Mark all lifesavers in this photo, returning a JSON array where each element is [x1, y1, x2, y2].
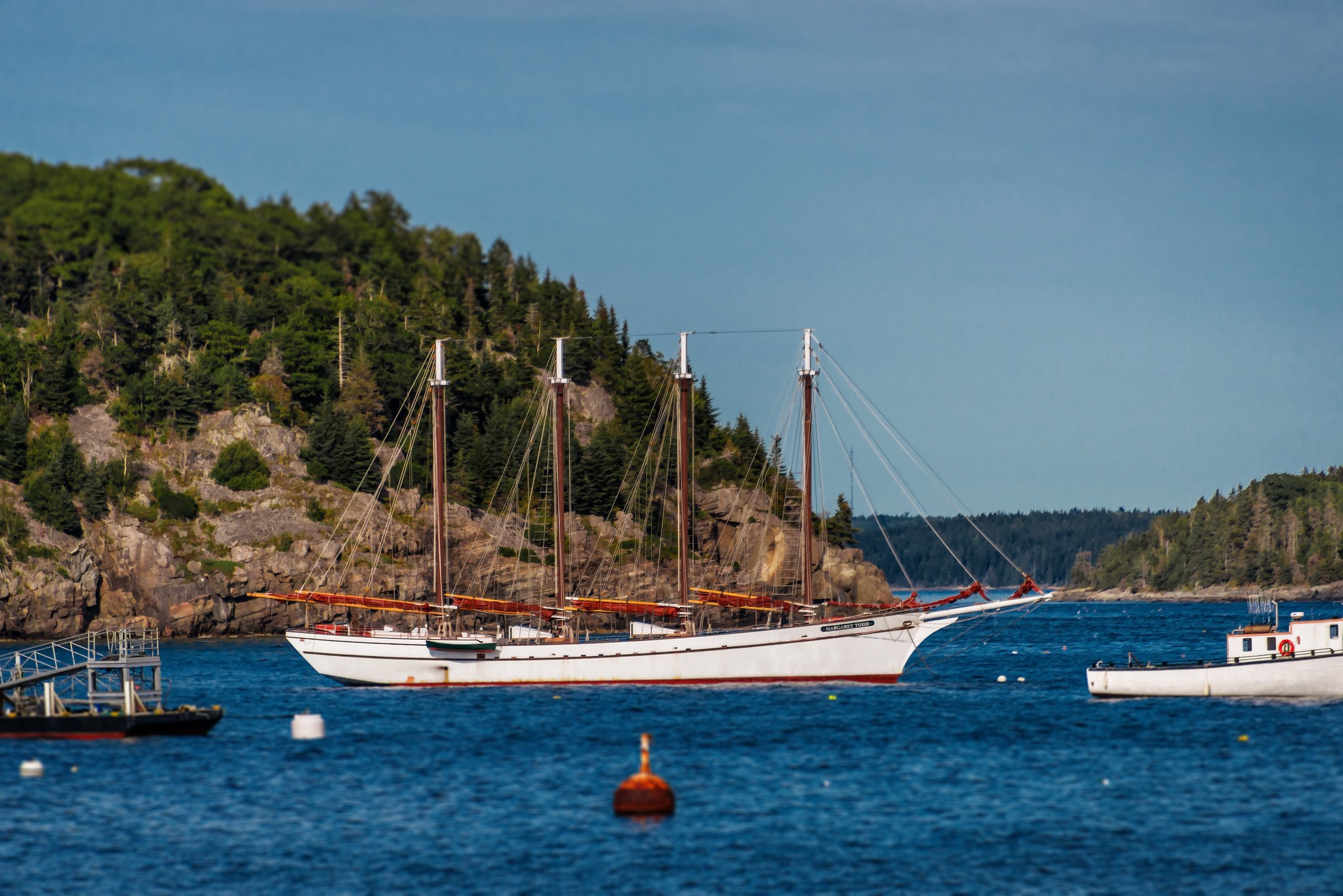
[[1278, 640, 1294, 656]]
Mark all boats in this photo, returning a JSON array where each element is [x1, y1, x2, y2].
[[1086, 600, 1342, 697], [425, 639, 497, 653], [0, 701, 226, 740]]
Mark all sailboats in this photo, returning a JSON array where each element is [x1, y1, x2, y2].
[[244, 329, 1057, 684]]
